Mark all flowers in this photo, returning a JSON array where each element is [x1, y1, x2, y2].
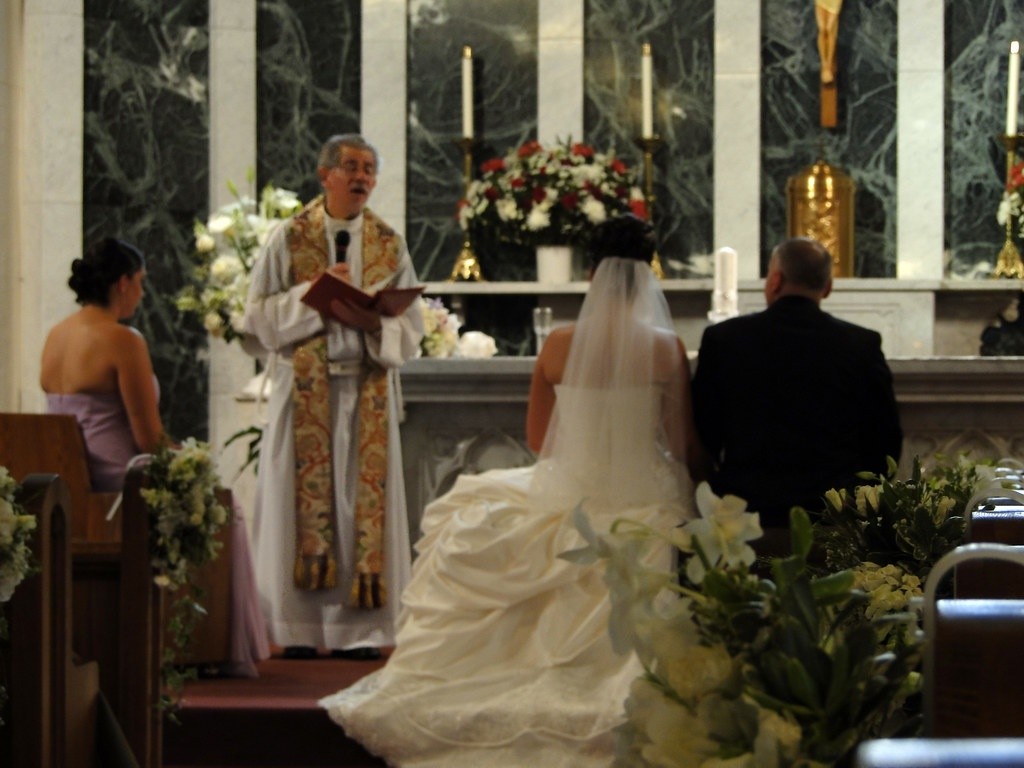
[[0, 461, 44, 647], [418, 299, 499, 357], [453, 134, 652, 263], [577, 449, 1020, 768], [170, 162, 303, 341], [138, 435, 232, 732]]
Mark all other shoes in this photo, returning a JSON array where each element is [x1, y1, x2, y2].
[[330, 648, 379, 662], [285, 647, 321, 660]]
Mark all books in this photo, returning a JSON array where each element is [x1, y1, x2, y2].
[[300, 273, 425, 337]]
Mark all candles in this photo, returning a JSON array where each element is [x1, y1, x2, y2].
[[461, 44, 474, 137], [1004, 40, 1019, 136], [713, 245, 736, 314], [640, 42, 652, 139]]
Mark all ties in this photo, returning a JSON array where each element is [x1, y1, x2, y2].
[[334, 229, 352, 265]]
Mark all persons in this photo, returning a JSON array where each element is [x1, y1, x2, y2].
[[39, 234, 267, 685], [251, 134, 429, 657], [318, 214, 713, 768], [689, 239, 903, 541]]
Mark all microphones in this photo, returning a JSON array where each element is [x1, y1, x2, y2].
[[334, 229, 350, 264]]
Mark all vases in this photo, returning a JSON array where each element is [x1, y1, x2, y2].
[[534, 246, 584, 283]]
